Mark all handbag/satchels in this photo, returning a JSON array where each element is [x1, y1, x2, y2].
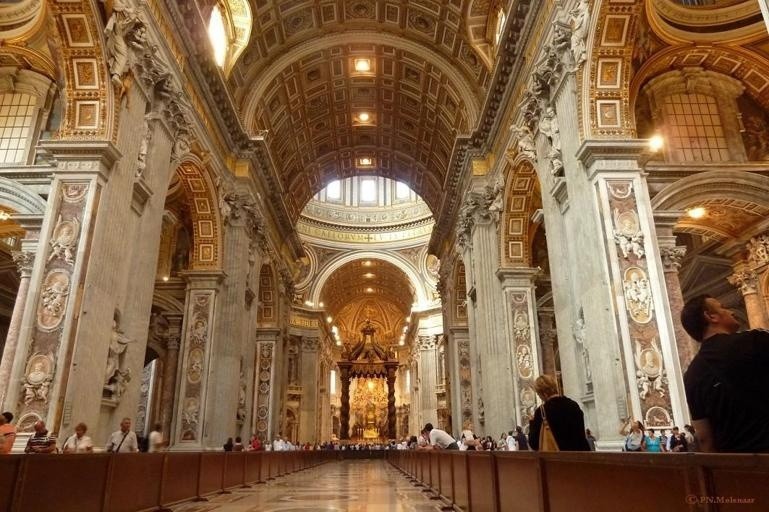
[[538, 404, 560, 452]]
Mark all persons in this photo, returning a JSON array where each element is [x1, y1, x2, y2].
[[220, 415, 694, 454], [528, 374, 591, 451], [679, 292, 768, 454], [24, 421, 58, 455], [42, 235, 76, 317], [0, 411, 17, 454], [612, 229, 633, 258], [104, 418, 140, 454], [21, 379, 50, 406], [62, 422, 93, 454], [146, 421, 169, 454], [653, 373, 666, 400], [507, 28, 577, 172], [636, 370, 652, 400], [628, 232, 646, 259]]
